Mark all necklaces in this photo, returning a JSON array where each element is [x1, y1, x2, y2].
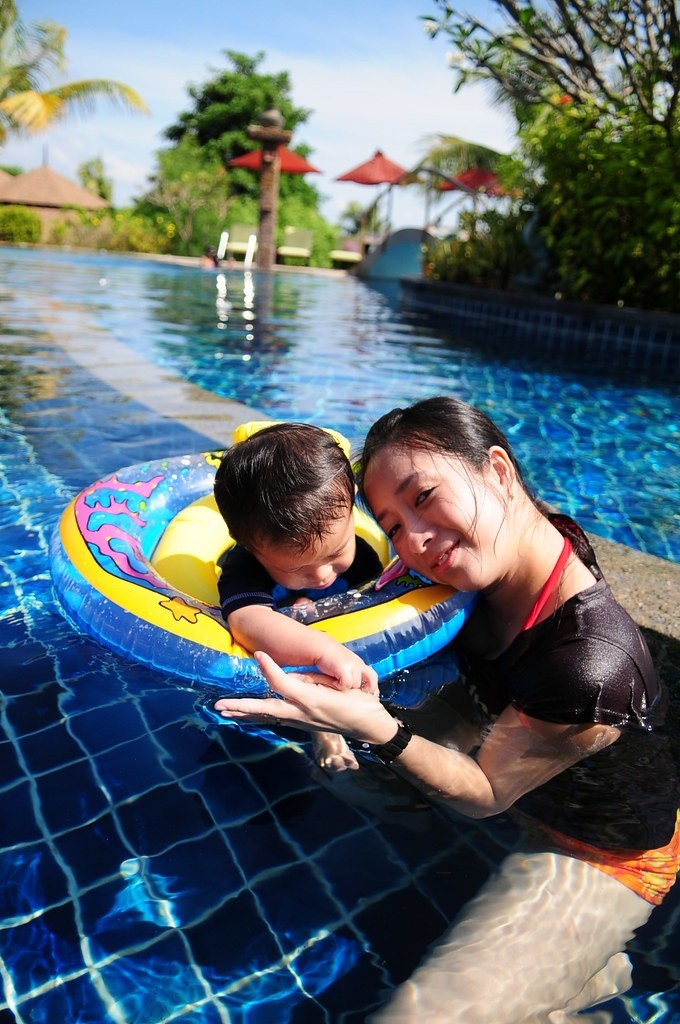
[[512, 538, 572, 637]]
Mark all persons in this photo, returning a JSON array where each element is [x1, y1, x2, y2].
[[215, 397, 680, 1024], [216, 423, 385, 772]]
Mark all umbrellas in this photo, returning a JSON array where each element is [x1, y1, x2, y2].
[[229, 142, 523, 239]]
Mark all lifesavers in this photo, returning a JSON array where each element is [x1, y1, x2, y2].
[[45, 446, 475, 689]]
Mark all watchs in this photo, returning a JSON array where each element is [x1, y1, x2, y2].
[[346, 700, 412, 764]]
